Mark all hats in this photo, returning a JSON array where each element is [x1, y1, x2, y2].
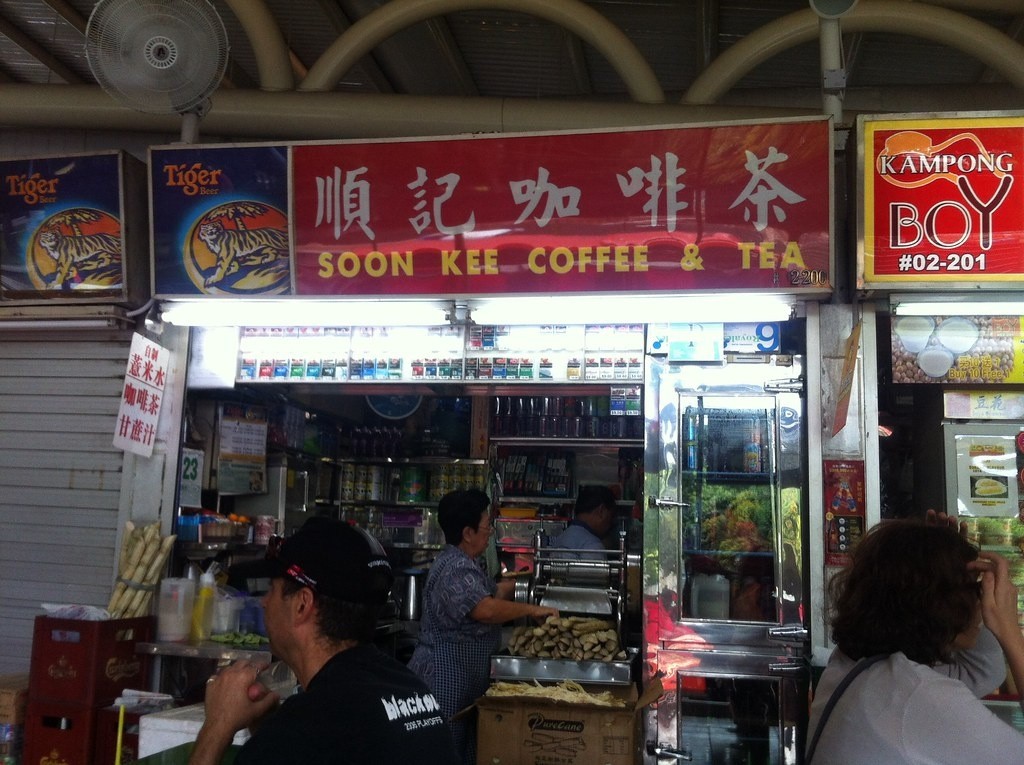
[[242, 517, 394, 608]]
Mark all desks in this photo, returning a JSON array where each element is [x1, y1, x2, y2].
[[138, 641, 274, 698]]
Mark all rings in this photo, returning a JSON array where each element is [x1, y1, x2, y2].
[[248, 663, 260, 675], [207, 678, 215, 684]]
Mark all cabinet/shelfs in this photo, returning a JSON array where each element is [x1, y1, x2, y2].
[[172, 389, 643, 574]]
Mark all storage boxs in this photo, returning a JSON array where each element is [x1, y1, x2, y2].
[[0, 611, 252, 765], [445, 676, 664, 765]]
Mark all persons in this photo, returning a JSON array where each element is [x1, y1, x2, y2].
[[805, 508, 1024, 765], [189, 517, 454, 765], [406, 489, 560, 765], [550, 485, 617, 561], [718, 541, 798, 765]]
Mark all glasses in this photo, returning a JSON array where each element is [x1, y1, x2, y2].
[[478, 517, 497, 531]]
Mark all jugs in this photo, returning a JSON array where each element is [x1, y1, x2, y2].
[[157, 578, 195, 643], [390, 574, 420, 621]]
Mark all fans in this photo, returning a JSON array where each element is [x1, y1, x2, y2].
[[366, 394, 424, 420], [85, 0, 231, 146]]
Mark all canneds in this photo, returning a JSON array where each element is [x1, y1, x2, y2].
[[683, 442, 698, 470], [743, 443, 761, 472], [492, 396, 633, 438], [683, 414, 697, 442], [341, 464, 484, 501]]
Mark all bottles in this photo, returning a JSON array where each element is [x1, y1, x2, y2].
[[201, 510, 231, 541]]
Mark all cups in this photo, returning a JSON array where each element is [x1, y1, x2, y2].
[[192, 588, 217, 641]]
